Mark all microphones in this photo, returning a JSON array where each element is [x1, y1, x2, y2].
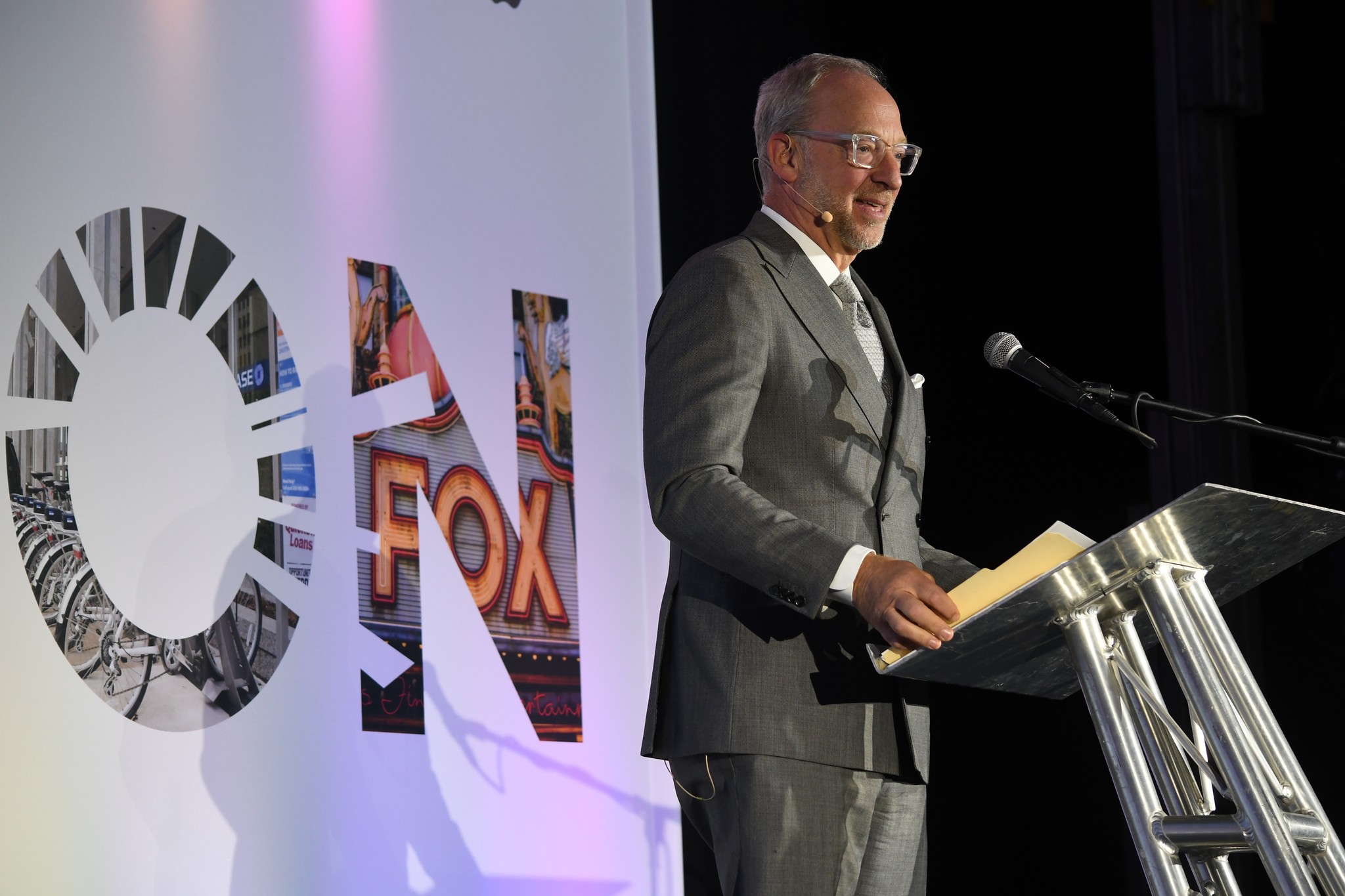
[[983, 331, 1120, 426], [779, 176, 834, 222]]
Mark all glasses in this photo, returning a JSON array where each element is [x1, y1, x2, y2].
[[786, 123, 922, 178]]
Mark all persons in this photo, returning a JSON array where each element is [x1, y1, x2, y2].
[[641, 53, 982, 896]]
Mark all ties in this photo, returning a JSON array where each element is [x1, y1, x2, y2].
[[831, 273, 884, 384]]
